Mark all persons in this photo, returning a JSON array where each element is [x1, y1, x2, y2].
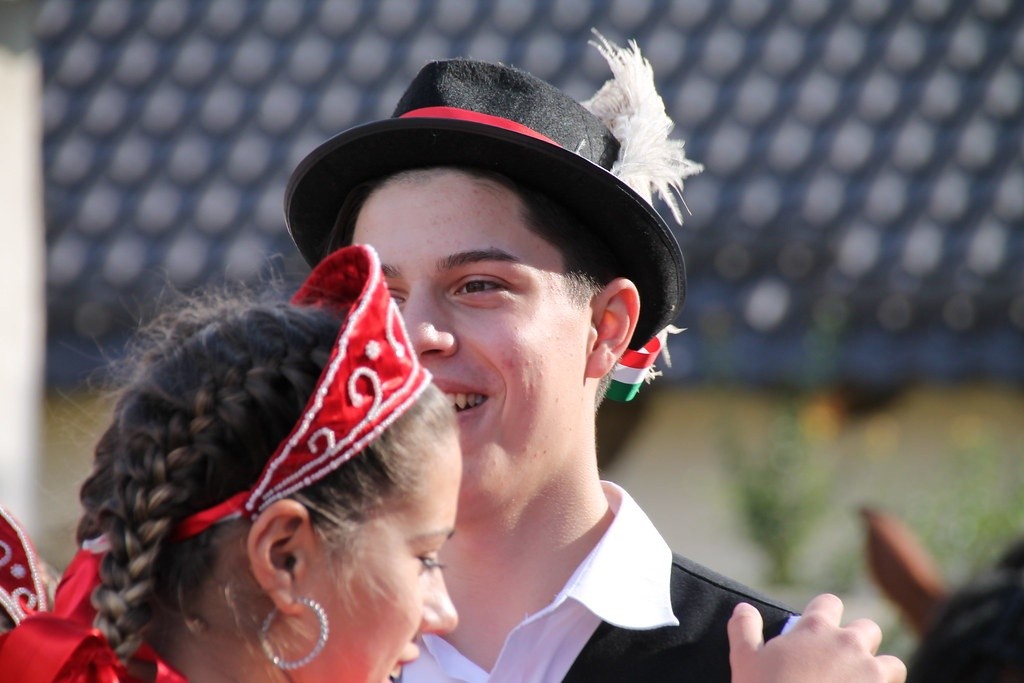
[[282, 57, 802, 683], [0, 246, 907, 683]]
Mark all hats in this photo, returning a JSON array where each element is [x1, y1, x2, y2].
[[284, 28, 703, 402]]
